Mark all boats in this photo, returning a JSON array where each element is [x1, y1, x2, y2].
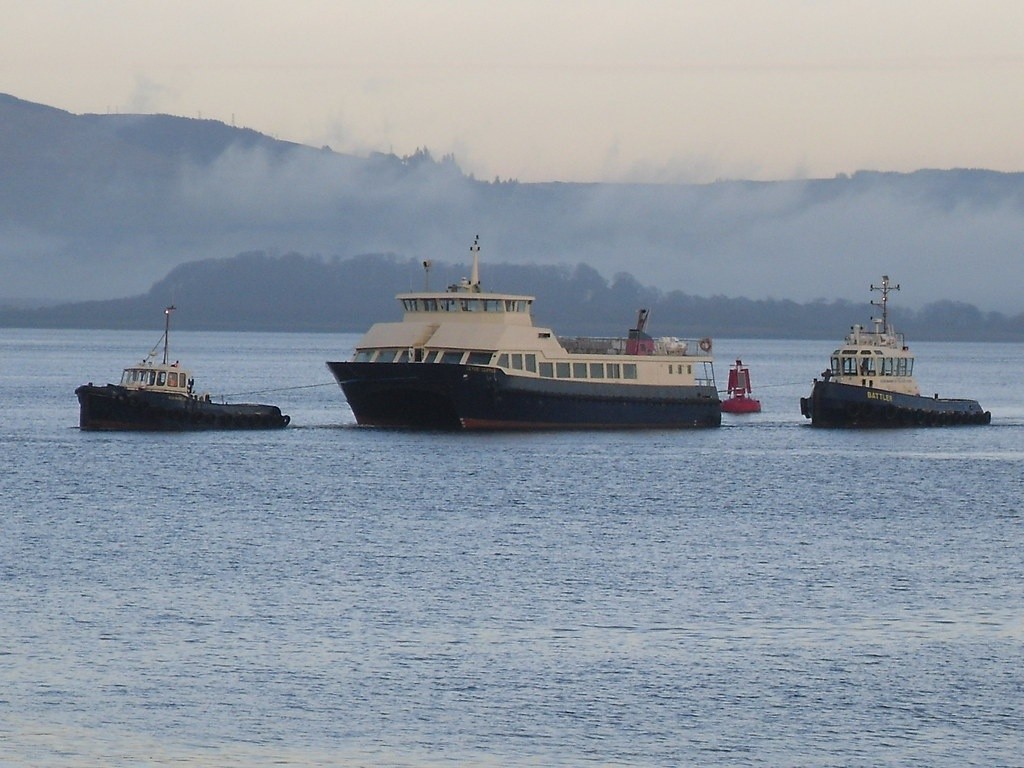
[[74, 304, 292, 431], [326, 233, 724, 430], [799, 275, 992, 428]]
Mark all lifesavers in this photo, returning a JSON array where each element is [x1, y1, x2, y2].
[[135, 400, 292, 427], [700, 339, 712, 351], [884, 404, 993, 426]]
